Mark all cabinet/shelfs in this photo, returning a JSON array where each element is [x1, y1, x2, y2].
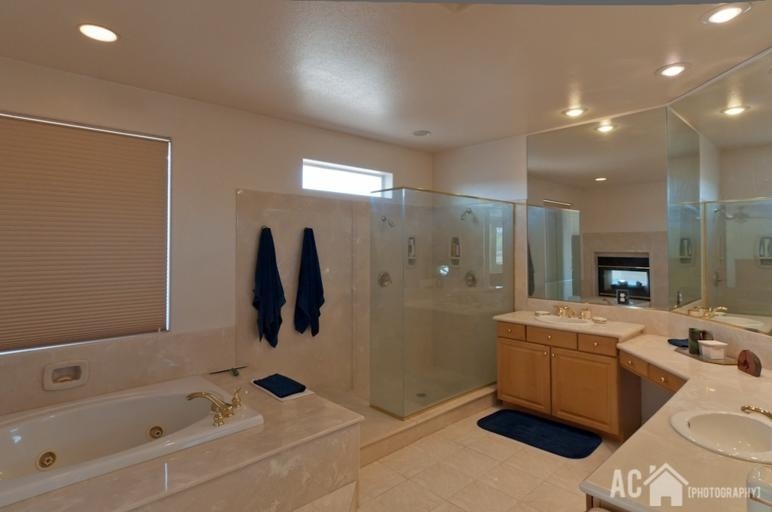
[[617, 332, 753, 396], [492, 308, 645, 445], [579, 378, 772, 512]]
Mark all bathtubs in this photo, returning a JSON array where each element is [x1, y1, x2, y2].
[[0, 375, 265, 509]]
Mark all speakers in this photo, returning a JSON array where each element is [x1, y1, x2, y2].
[[616, 290, 629, 304]]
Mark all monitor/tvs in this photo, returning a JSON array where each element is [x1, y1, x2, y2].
[[598, 266, 650, 301]]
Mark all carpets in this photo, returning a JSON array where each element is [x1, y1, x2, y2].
[[477, 408, 602, 459]]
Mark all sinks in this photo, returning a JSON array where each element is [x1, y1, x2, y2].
[[535, 316, 593, 326], [669, 410, 772, 464]]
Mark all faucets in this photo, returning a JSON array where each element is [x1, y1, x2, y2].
[[185, 385, 245, 425], [741, 404, 772, 422], [455, 204, 477, 226], [552, 303, 571, 318]]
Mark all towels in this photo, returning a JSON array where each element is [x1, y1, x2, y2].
[[253, 374, 306, 398], [250, 226, 287, 350], [293, 225, 325, 338]]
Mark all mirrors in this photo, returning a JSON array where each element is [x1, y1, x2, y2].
[[527, 45, 772, 341]]
